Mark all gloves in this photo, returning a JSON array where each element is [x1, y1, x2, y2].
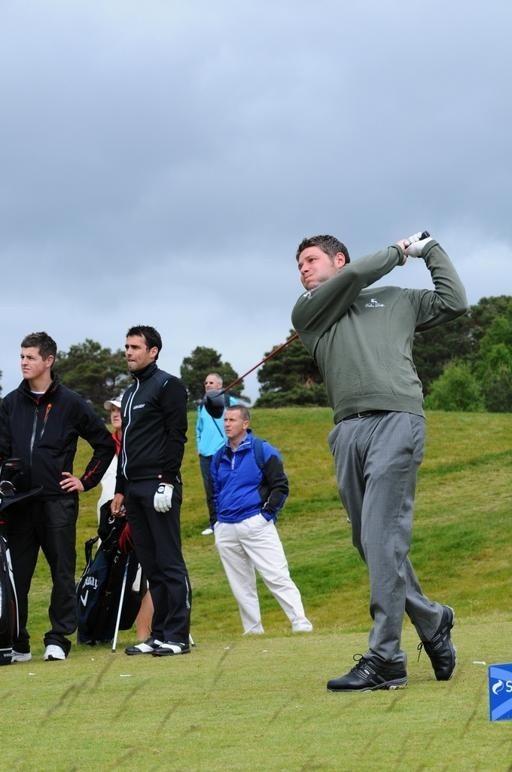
[[402, 230, 436, 259], [152, 481, 175, 513]]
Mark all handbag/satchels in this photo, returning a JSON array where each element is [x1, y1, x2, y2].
[[0, 533, 21, 668], [75, 498, 150, 647]]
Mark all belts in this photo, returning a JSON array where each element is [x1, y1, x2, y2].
[[339, 410, 387, 422]]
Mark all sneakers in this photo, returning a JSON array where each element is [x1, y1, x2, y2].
[[125, 637, 166, 655], [326, 654, 410, 693], [40, 642, 66, 662], [151, 640, 190, 656], [201, 525, 215, 536], [6, 647, 34, 663], [421, 604, 456, 682]]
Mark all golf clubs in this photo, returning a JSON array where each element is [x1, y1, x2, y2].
[[204, 229, 427, 418]]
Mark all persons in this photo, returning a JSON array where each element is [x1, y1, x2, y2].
[[109, 323, 193, 657], [194, 371, 240, 536], [0, 330, 118, 664], [98, 389, 155, 643], [205, 404, 316, 635], [290, 232, 470, 693]]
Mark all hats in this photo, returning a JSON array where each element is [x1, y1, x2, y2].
[[102, 393, 124, 411]]
